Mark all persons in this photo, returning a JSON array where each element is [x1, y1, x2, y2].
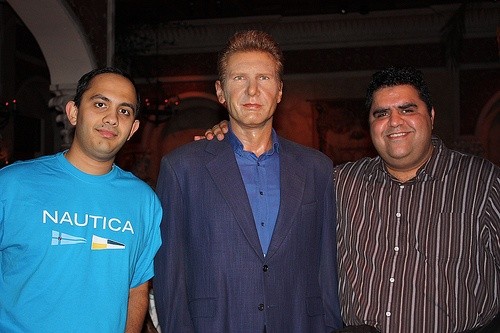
[[151, 29, 345, 333], [193, 69, 500, 333], [0, 67, 163, 333]]
